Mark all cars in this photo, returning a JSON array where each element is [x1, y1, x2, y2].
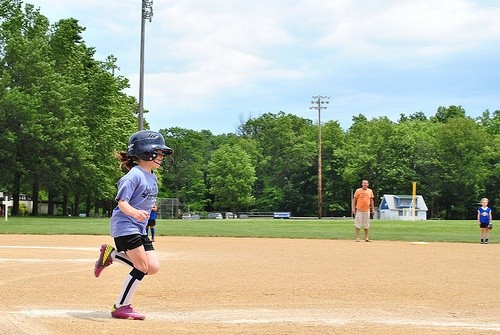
[[182, 212, 200, 220], [228, 213, 237, 219], [213, 213, 223, 220]]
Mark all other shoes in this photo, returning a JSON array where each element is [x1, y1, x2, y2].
[[480, 239, 483, 244], [365, 239, 369, 241], [485, 239, 488, 244]]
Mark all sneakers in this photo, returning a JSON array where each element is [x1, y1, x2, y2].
[[94, 244, 115, 277], [111, 303, 145, 320]]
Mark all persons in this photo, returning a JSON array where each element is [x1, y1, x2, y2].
[[146, 203, 157, 242], [94, 131, 173, 320], [352, 180, 375, 242], [477, 198, 492, 244]]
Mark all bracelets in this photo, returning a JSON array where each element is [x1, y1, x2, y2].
[[372, 209, 374, 211]]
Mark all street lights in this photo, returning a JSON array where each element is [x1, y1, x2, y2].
[[310, 94, 330, 219], [137, 0, 155, 131]]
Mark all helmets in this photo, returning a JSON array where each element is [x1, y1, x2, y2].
[[128, 130, 174, 161]]
